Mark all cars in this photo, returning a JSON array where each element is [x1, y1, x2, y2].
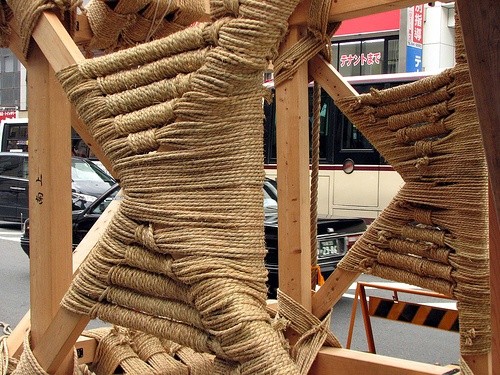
[[1, 150, 120, 227], [23, 178, 367, 302]]
